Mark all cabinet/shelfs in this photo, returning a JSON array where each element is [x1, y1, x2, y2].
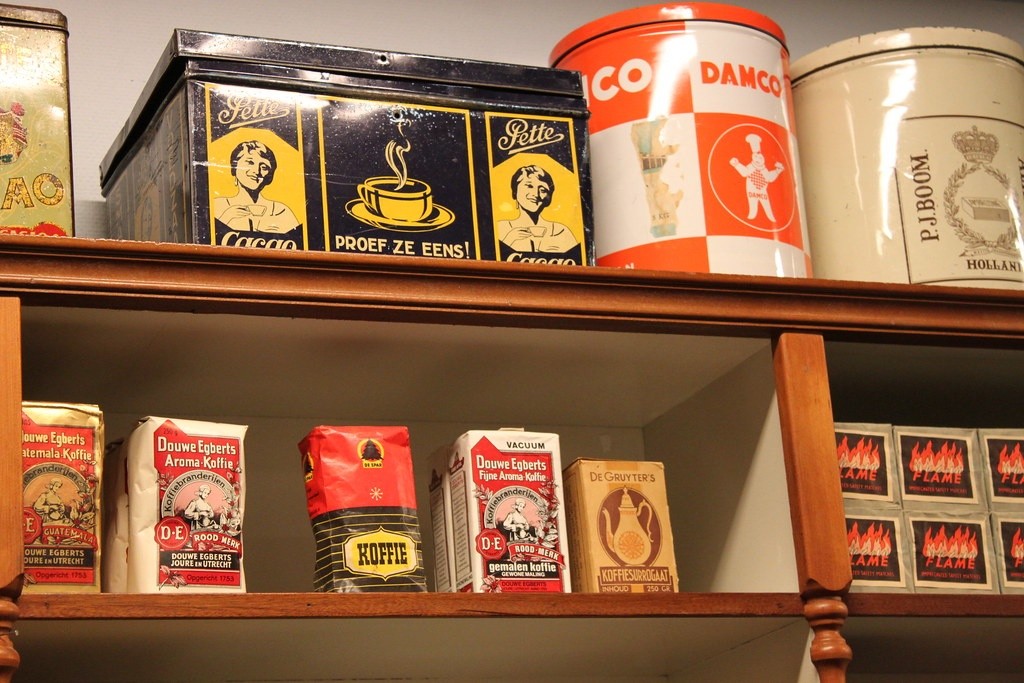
[[0, 232, 1024, 683]]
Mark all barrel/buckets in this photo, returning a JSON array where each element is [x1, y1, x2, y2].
[[789, 27, 1024, 291], [549, 2, 813, 279]]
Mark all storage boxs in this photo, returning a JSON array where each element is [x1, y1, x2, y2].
[[101, 435, 129, 592], [298, 424, 428, 592], [990, 512, 1024, 595], [892, 425, 988, 512], [100, 28, 594, 263], [0, 4, 77, 237], [426, 460, 457, 593], [126, 415, 250, 593], [833, 421, 903, 510], [844, 508, 915, 595], [445, 428, 573, 594], [977, 428, 1024, 512], [21, 399, 107, 595], [561, 457, 681, 593], [904, 512, 1001, 596]]
